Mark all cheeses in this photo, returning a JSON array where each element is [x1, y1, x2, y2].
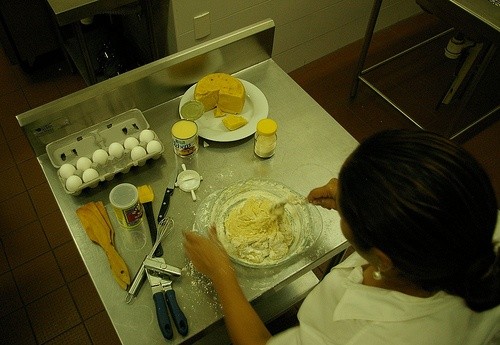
[[194, 73, 248, 130]]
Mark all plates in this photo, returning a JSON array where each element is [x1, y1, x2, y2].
[[178, 78, 269, 142]]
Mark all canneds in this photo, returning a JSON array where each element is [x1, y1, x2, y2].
[[109, 183, 144, 229], [171, 100, 204, 158]]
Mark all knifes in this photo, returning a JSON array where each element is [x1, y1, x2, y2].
[[157, 168, 178, 225]]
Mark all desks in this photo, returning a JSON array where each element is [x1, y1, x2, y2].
[[36, 57, 360, 345], [47, 0, 160, 86], [351, 0, 500, 142]]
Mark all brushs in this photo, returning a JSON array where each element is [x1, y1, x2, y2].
[[138, 185, 164, 257]]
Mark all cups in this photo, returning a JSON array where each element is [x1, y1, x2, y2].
[[109, 183, 144, 228], [171, 119, 199, 159]]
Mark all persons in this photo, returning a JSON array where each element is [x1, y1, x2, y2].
[[182, 129, 499, 343]]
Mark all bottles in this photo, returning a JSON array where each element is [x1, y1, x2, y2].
[[253, 118, 278, 159]]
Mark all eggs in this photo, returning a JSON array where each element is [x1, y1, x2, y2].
[[59, 129, 162, 191]]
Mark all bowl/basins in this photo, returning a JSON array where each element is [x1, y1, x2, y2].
[[191, 177, 323, 269]]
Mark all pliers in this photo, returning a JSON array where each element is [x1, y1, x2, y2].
[[144, 258, 188, 339]]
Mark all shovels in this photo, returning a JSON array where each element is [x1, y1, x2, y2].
[[75, 201, 131, 288]]
